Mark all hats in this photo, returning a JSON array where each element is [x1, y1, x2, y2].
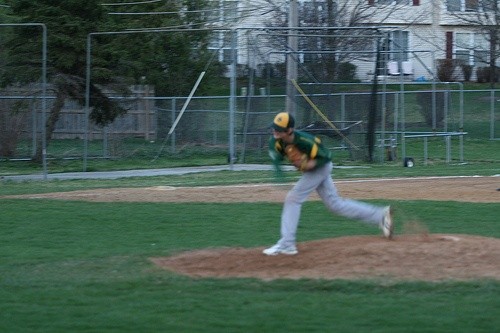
[[266, 111, 296, 132]]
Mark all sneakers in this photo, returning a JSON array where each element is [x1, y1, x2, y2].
[[261, 238, 299, 256], [379, 204, 394, 239]]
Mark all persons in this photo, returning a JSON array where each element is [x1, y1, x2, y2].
[[261, 112, 392, 256]]
